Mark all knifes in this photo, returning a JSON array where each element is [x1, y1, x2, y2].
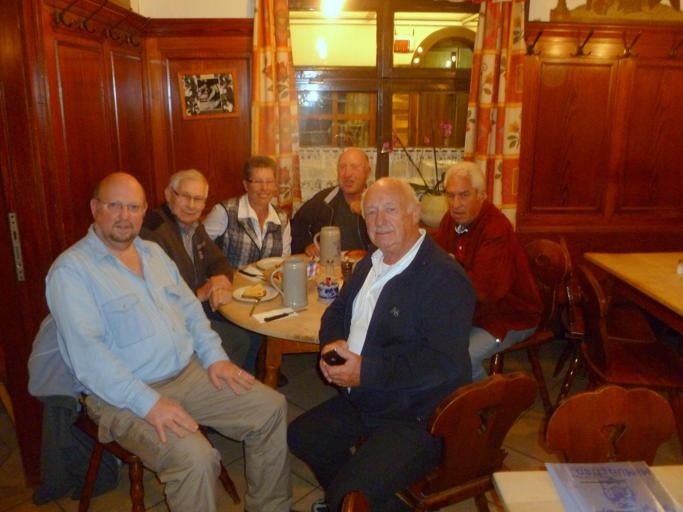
[[263, 307, 308, 324]]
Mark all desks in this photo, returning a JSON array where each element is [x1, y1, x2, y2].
[[492, 465, 682, 512], [585, 250, 683, 344]]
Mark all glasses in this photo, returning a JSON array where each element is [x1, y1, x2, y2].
[[95, 196, 146, 214], [247, 178, 275, 186], [169, 185, 207, 202]]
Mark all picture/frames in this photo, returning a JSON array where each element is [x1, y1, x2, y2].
[[176, 69, 243, 121]]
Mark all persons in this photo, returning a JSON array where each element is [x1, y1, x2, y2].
[[45, 172, 292, 512], [431, 162, 547, 380], [141, 169, 252, 368], [291, 147, 379, 257], [202, 155, 292, 388], [287, 176, 475, 512]]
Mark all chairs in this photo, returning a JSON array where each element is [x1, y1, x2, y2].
[[398, 372, 542, 512], [555, 236, 596, 375], [559, 265, 679, 394], [75, 413, 242, 512], [490, 241, 560, 375], [545, 385, 678, 466]]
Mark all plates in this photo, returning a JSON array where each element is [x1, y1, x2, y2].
[[256, 256, 282, 272], [230, 283, 280, 302]]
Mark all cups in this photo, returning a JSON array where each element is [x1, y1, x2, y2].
[[313, 226, 342, 266], [270, 255, 307, 308]]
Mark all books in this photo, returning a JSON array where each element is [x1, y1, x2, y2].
[[542, 462, 681, 508]]
